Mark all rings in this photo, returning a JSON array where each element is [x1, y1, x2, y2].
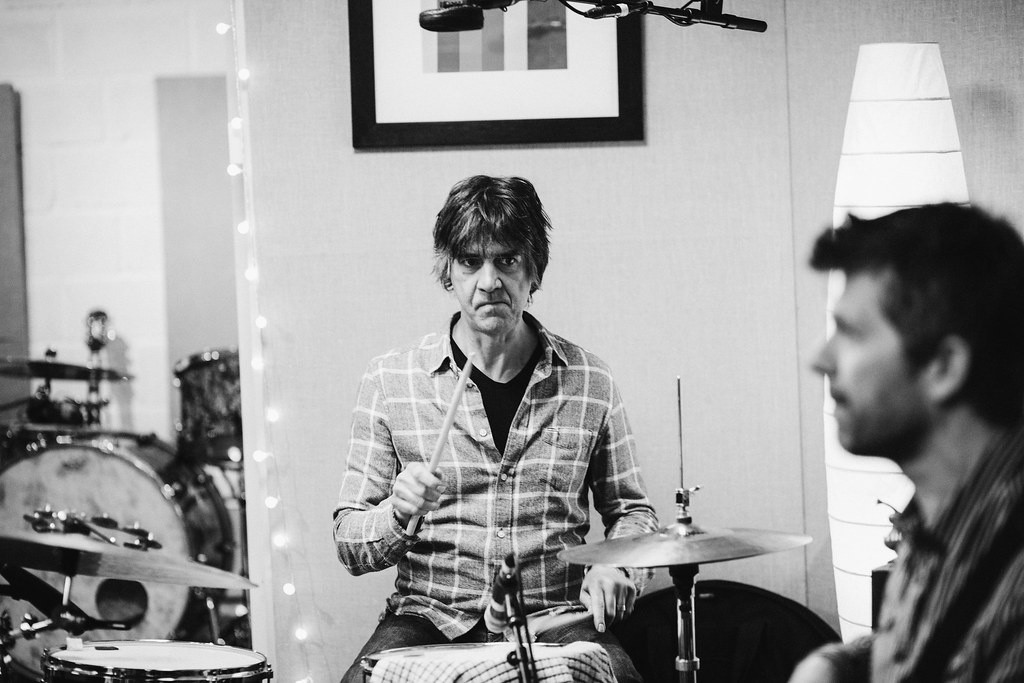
[[617, 603, 626, 611]]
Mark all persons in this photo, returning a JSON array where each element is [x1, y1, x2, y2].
[[331, 173, 662, 683], [786, 199, 1023, 683]]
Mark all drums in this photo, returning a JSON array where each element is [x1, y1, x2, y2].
[[174, 344, 240, 460], [0, 428, 235, 680], [362, 642, 611, 683], [38, 639, 274, 683]]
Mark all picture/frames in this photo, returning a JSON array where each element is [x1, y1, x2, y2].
[[347, 0, 644, 149]]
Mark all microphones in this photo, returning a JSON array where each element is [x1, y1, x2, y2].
[[484, 553, 516, 634], [419, 0, 518, 32]]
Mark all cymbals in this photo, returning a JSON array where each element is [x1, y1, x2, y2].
[[0, 357, 134, 382], [558, 522, 814, 570], [0, 537, 260, 589]]
